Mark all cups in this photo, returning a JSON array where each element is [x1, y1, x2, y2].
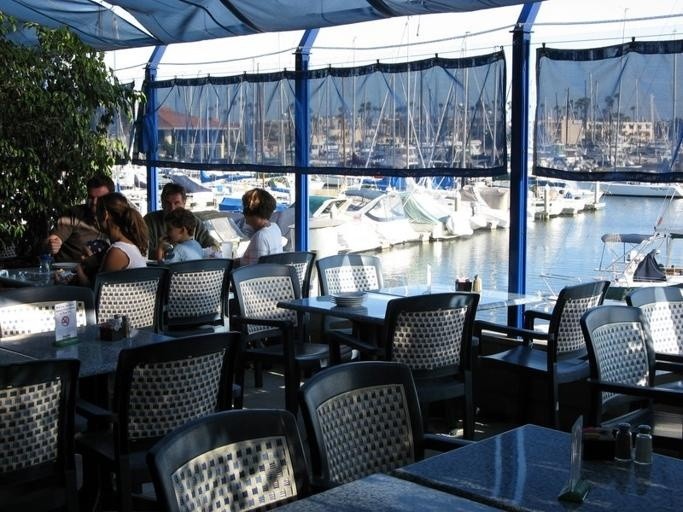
[[232, 236, 239, 250], [221, 242, 232, 259]]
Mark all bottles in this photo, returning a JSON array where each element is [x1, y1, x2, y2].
[[614, 423, 632, 461], [454, 274, 481, 292], [579, 427, 618, 463], [97, 314, 130, 341]]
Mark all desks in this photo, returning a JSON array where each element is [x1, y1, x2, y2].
[[393, 423, 683, 512]]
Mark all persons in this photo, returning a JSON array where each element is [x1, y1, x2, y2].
[[42, 174, 284, 315]]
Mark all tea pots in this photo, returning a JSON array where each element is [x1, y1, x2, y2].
[[36, 254, 50, 284]]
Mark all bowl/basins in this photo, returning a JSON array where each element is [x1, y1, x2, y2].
[[52, 262, 77, 269], [18, 271, 55, 280], [330, 292, 367, 306]]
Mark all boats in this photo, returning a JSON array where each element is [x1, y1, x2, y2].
[[536, 184, 682, 320]]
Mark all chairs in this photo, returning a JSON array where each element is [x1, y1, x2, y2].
[[0, 250, 499, 512], [469, 280, 683, 461]]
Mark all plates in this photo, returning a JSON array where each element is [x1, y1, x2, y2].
[[633, 424, 654, 465]]
